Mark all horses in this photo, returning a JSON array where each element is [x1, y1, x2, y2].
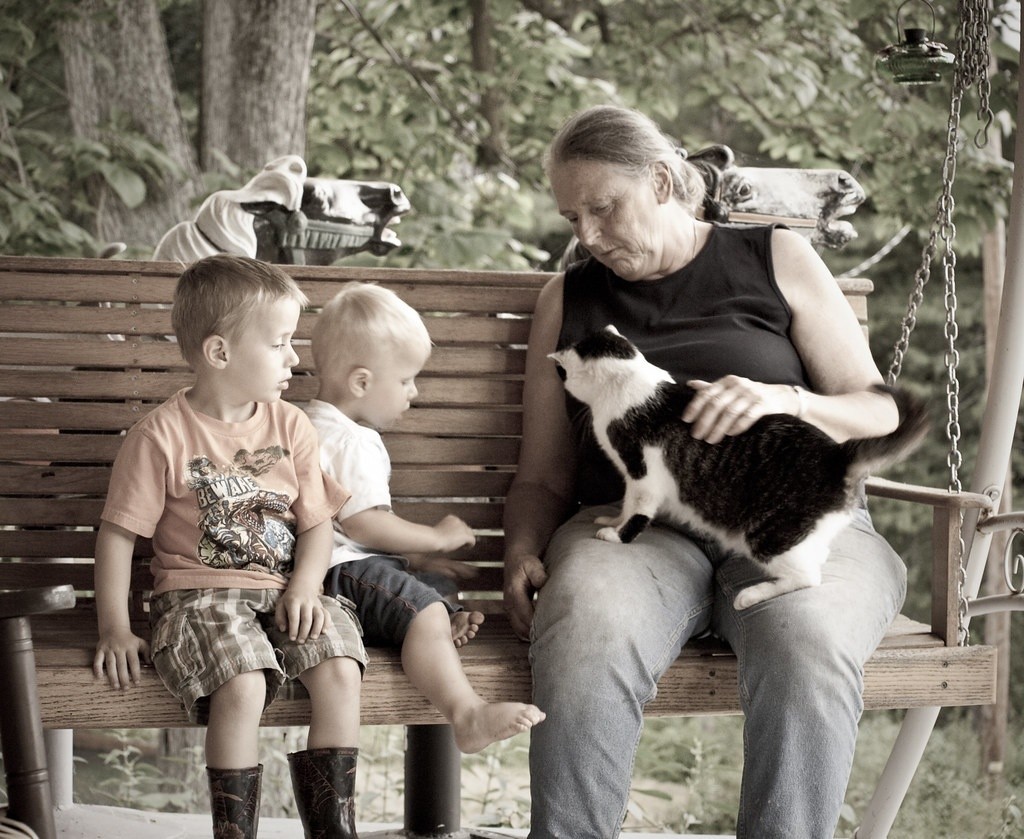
[[556, 143, 867, 272]]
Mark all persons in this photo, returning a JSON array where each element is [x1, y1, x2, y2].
[[503, 103, 908, 837], [95, 255, 368, 839], [303, 283, 546, 754]]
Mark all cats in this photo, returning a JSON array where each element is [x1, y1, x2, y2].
[[545, 322, 935, 613]]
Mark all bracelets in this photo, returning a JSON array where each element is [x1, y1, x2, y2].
[[790, 381, 808, 419]]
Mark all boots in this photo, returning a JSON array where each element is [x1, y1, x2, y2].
[[203, 763, 262, 838], [286, 747, 359, 839]]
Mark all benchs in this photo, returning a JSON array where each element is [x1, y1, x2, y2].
[[0, 250, 997, 839]]
